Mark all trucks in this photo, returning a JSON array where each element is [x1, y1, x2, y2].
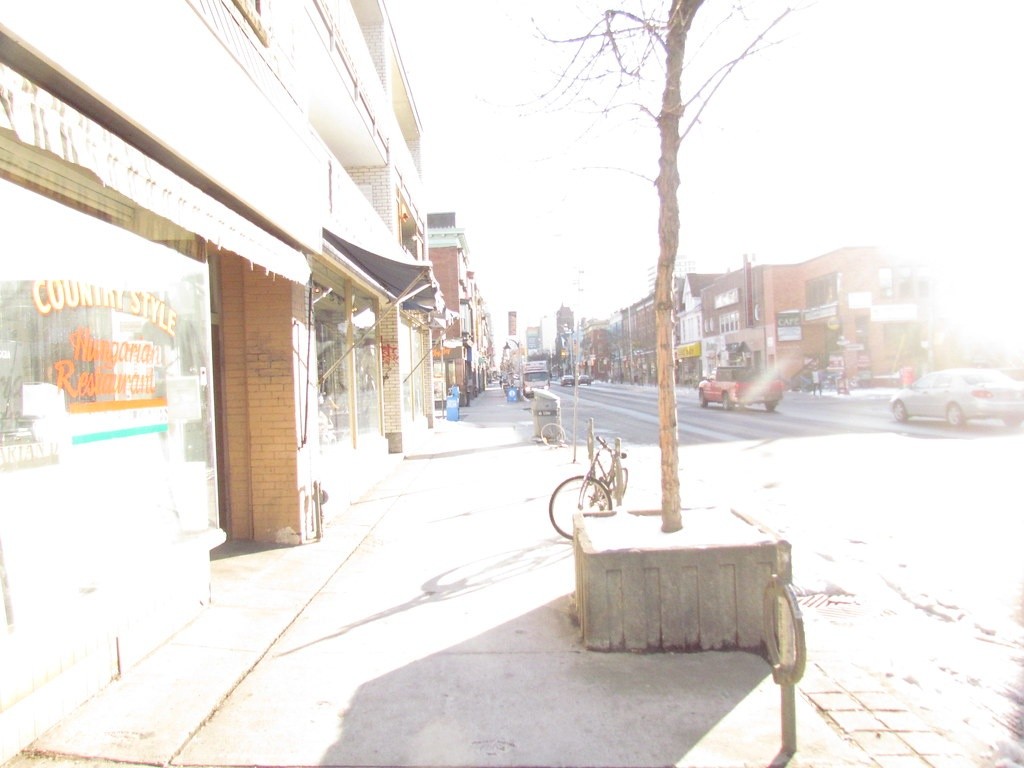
[[521, 361, 553, 398]]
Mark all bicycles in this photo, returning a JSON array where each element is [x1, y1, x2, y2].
[[548, 435, 629, 540], [536, 423, 568, 449]]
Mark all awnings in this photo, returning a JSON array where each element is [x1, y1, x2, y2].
[[318, 229, 458, 384]]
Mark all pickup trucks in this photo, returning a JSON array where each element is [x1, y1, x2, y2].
[[698, 366, 783, 411]]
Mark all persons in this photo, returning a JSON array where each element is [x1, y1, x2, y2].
[[899, 363, 912, 385], [811, 367, 823, 395]]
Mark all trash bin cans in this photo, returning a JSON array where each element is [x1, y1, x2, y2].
[[531, 387, 561, 439], [446, 395, 459, 421], [506, 387, 518, 401]]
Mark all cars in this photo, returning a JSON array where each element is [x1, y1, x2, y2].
[[891, 367, 1024, 427], [513, 374, 519, 380], [578, 375, 591, 386], [560, 375, 575, 387], [782, 373, 814, 393]]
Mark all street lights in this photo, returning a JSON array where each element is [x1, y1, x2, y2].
[[540, 316, 547, 360], [623, 306, 634, 385]]
